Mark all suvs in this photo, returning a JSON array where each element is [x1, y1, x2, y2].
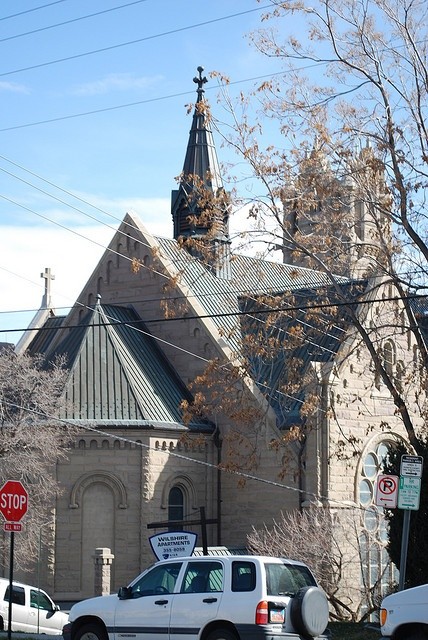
[[380, 584, 428, 640], [68, 556, 329, 640], [0, 580, 68, 635]]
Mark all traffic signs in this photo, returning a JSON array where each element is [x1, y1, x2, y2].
[[398, 477, 421, 510], [400, 454, 423, 477]]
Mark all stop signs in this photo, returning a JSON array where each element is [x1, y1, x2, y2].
[[0, 481, 28, 522]]
